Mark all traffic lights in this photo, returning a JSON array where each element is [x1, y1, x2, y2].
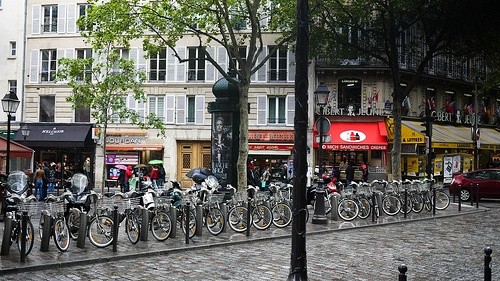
[[420, 116, 429, 137]]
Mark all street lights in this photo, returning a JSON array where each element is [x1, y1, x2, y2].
[[2, 91, 20, 176], [312, 78, 331, 223]]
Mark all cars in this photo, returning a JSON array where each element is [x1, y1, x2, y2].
[[449, 169, 500, 203]]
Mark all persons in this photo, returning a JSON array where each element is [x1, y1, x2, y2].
[[33, 163, 45, 200], [345, 163, 354, 182], [362, 164, 369, 182], [150, 164, 158, 189], [41, 161, 50, 197], [247, 156, 258, 197], [117, 169, 125, 192], [339, 157, 348, 169], [158, 163, 165, 186]]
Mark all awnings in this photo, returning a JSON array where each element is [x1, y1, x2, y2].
[[13, 122, 93, 147], [312, 117, 499, 152]]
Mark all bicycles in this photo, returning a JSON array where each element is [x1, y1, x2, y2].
[[38, 193, 71, 252], [253, 184, 293, 228], [285, 184, 310, 224], [193, 185, 225, 236], [132, 186, 173, 242], [159, 182, 198, 239], [312, 178, 449, 221], [65, 192, 115, 248], [212, 183, 253, 232], [100, 191, 141, 245], [229, 184, 273, 231], [8, 193, 34, 254]]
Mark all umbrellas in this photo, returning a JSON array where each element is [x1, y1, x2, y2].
[[134, 163, 149, 168], [185, 167, 215, 177], [115, 163, 127, 169], [148, 159, 164, 164]]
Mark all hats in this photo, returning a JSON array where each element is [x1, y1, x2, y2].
[[246, 156, 368, 173]]
